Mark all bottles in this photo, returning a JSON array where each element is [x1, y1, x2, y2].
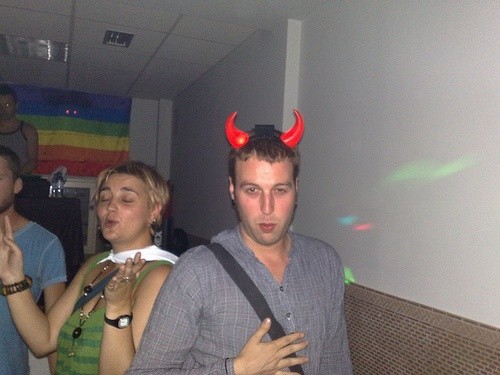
[[52, 172, 63, 198]]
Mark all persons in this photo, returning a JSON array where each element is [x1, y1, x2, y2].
[[0, 162, 179, 375], [0, 145, 67, 375], [124, 108, 354, 375], [0, 85, 39, 217]]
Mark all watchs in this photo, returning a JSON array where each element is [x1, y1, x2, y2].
[[103, 313, 133, 328]]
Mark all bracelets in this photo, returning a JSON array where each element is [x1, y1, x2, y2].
[[0, 274, 34, 295]]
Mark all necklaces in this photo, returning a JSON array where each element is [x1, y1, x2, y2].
[[67, 262, 113, 357]]
